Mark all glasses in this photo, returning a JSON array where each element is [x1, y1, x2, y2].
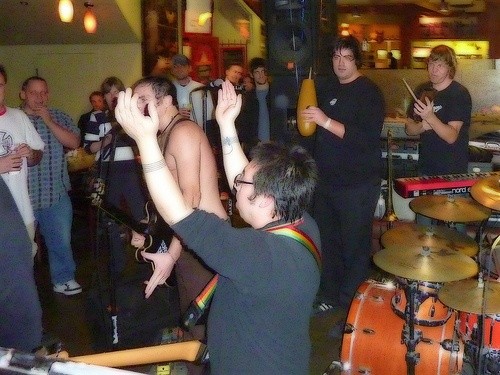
[[233, 174, 254, 185], [333, 55, 356, 62]]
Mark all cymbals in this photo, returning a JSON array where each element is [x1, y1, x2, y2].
[[470, 175, 500, 213], [380, 223, 479, 257], [436, 278, 500, 315], [372, 243, 479, 283], [409, 194, 492, 223]]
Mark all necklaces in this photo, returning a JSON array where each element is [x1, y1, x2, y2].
[[160, 112, 181, 135]]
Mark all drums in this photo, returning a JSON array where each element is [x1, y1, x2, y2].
[[475, 244, 500, 280], [454, 310, 500, 363], [338, 269, 471, 375]]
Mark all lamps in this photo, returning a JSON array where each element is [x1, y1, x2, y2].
[[57, 0, 75, 24], [81, 0, 99, 35]]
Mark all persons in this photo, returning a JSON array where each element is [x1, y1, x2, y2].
[[301, 34, 385, 313], [20, 76, 82, 294], [0, 174, 41, 352], [115, 80, 322, 375], [404, 45, 472, 234], [79, 56, 288, 338], [0, 65, 39, 257]]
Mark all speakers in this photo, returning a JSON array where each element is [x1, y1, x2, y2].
[[192, 78, 223, 91], [269, 74, 342, 155], [265, 0, 340, 74]]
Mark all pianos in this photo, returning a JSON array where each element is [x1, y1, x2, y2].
[[392, 171, 500, 231]]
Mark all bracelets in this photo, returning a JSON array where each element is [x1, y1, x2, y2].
[[167, 250, 177, 264], [324, 118, 331, 129]]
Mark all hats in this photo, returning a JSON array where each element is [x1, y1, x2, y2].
[[172, 54, 188, 64]]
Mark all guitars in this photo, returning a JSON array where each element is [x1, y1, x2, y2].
[[83, 170, 178, 291], [30, 332, 210, 369]]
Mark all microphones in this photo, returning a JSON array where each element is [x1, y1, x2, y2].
[[235, 85, 246, 91]]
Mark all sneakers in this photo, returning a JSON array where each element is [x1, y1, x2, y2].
[[314, 302, 337, 313], [329, 320, 346, 338], [53, 278, 82, 295]]
